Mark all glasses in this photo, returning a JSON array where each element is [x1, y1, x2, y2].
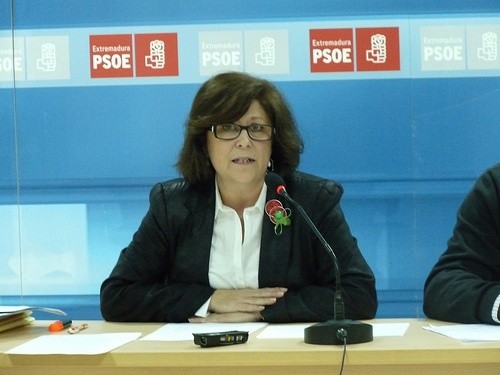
[[205, 122, 276, 141]]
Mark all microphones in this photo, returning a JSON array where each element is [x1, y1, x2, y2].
[[265, 172, 344, 323]]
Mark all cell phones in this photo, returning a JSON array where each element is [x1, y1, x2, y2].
[[192, 330, 248, 347]]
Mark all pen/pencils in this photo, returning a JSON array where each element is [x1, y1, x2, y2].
[[49, 318, 72, 333], [68, 324, 88, 334]]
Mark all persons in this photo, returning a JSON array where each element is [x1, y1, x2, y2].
[[423, 162, 500, 325], [99, 72, 378, 323]]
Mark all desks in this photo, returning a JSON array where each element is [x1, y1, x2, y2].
[[0, 319, 500, 375]]
